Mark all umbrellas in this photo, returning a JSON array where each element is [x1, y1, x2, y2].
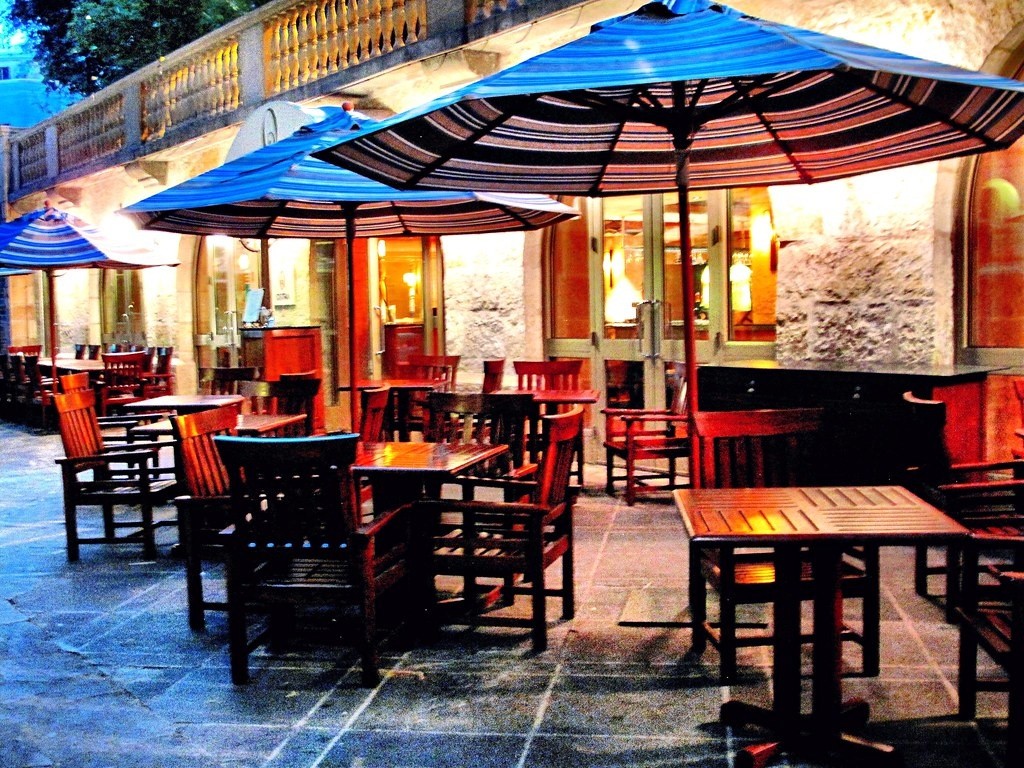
[[113, 102, 582, 432], [0, 201, 182, 396], [311, 0, 1024, 496]]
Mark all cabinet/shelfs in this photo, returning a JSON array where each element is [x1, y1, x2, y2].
[[240, 325, 327, 435], [382, 323, 423, 380]]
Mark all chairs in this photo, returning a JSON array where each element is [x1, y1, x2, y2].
[[0, 343, 1024, 768]]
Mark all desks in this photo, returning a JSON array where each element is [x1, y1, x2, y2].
[[490, 390, 602, 463], [338, 378, 451, 443], [123, 395, 244, 479], [351, 443, 509, 651], [37, 359, 139, 381], [668, 488, 972, 768]]
